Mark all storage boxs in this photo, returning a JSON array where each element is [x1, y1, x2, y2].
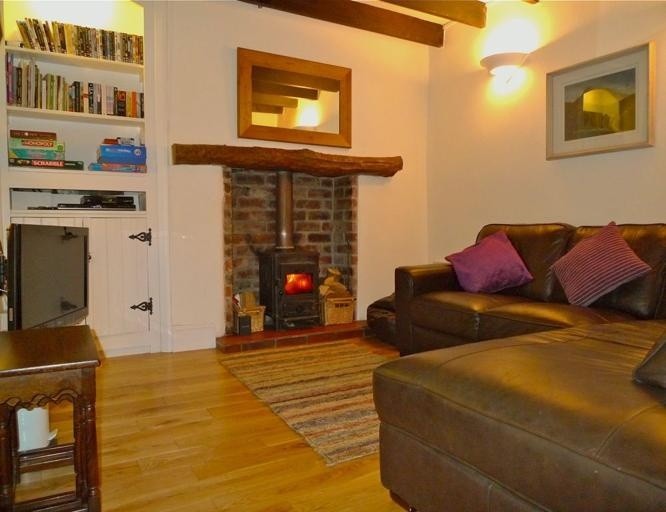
[[321, 295, 356, 325]]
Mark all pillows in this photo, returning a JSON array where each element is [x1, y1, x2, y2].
[[549, 221, 652, 308], [563, 223, 665, 319], [474, 223, 574, 302], [632, 329, 665, 389], [443, 225, 532, 292]]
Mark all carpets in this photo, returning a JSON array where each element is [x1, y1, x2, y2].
[[217, 338, 396, 468]]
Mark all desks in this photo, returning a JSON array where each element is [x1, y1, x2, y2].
[[0, 324, 103, 511]]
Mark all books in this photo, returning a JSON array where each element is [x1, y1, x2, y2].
[[2, 16, 147, 213]]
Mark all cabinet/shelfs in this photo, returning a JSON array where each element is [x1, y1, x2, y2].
[[0, 0, 152, 213], [0, 213, 152, 357]]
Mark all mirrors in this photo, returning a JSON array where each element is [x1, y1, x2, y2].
[[236, 46, 353, 149]]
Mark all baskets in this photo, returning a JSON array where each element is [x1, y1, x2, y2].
[[233, 305, 266, 335], [318, 297, 357, 326]]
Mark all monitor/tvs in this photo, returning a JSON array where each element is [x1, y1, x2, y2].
[[8, 224, 89, 329]]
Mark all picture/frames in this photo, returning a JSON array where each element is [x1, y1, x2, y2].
[[545, 40, 657, 161]]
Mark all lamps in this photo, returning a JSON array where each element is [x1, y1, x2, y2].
[[479, 52, 529, 76]]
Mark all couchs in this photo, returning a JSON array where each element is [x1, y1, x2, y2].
[[372, 220, 666, 512]]
